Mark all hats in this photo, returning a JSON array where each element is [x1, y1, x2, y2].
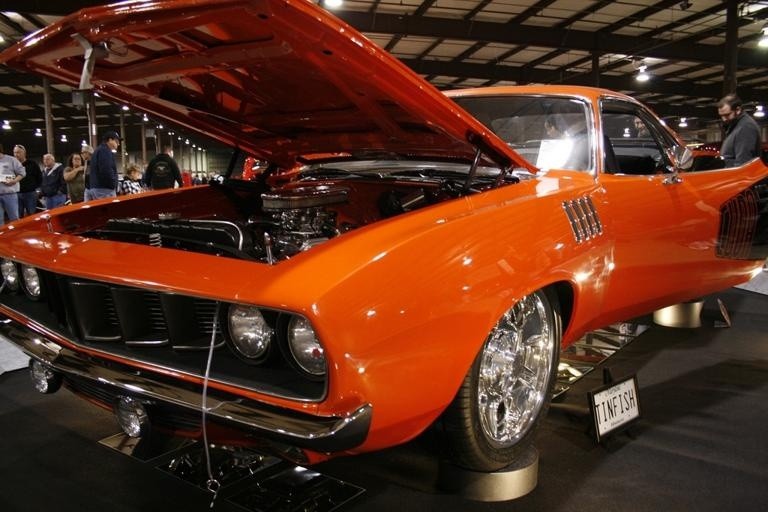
[[129, 165, 143, 174], [105, 131, 124, 141], [82, 145, 93, 154]]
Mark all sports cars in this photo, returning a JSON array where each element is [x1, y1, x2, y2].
[[0, 2, 767, 473]]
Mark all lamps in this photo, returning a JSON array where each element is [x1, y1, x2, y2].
[[753, 106, 765, 117], [758, 22, 768, 47], [635, 62, 650, 81], [1, 88, 207, 156]]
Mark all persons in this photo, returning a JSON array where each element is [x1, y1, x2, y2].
[[89, 131, 124, 200], [145, 140, 183, 191], [544, 114, 572, 139], [0, 144, 224, 226], [715, 93, 762, 167], [632, 114, 653, 139]]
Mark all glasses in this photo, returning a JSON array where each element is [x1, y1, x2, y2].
[[15, 145, 24, 150]]
